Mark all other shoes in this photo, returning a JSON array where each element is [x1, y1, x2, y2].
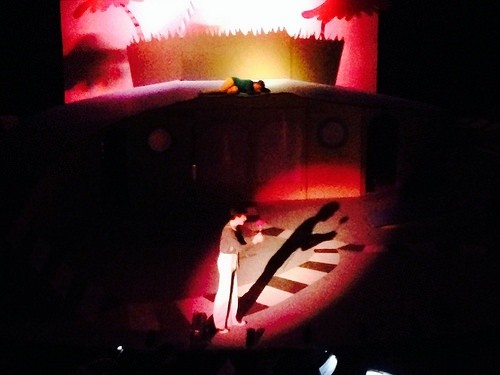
[[226, 320, 247, 328], [217, 327, 229, 335]]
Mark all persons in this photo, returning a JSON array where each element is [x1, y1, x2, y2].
[[213, 203, 265, 333], [197, 77, 265, 98]]
